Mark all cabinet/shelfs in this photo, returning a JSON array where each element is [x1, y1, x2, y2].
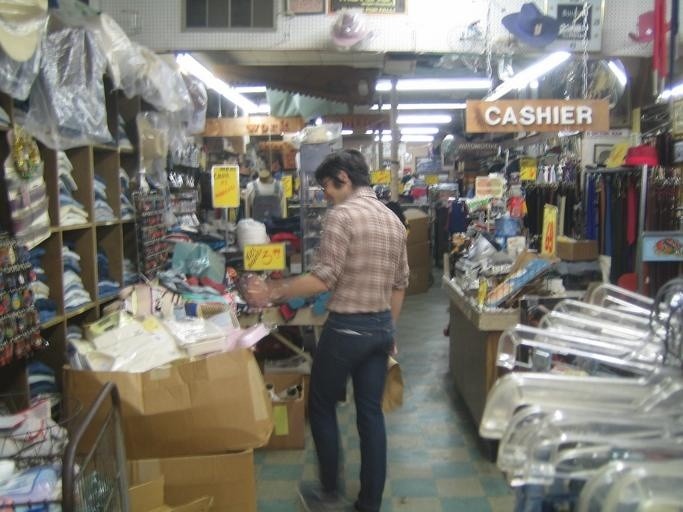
[[0, 95, 141, 412]]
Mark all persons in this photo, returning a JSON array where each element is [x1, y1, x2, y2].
[[242, 147, 410, 512], [246, 168, 289, 224]]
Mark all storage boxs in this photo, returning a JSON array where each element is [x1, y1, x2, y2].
[[404, 206, 431, 295], [60, 343, 275, 457], [257, 366, 307, 450], [83, 448, 256, 511]]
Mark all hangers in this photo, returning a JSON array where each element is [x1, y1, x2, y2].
[[477, 274, 683, 511]]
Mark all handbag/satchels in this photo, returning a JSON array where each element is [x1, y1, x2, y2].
[[381, 354, 402, 412]]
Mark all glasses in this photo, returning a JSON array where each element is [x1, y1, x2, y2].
[[321, 177, 333, 193]]
